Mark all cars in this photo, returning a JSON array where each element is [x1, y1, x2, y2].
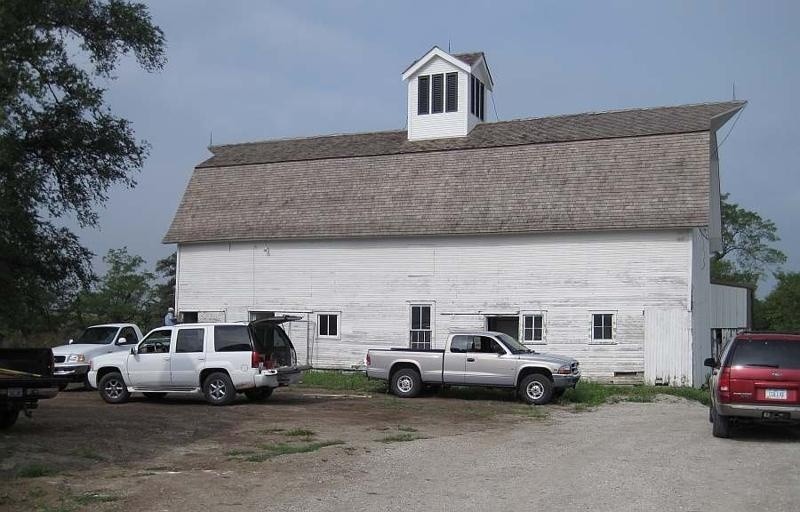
[[704, 333, 800, 438]]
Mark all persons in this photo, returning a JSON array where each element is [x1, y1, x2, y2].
[[164, 307, 177, 327]]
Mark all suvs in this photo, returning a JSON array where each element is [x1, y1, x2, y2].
[[89, 315, 313, 407]]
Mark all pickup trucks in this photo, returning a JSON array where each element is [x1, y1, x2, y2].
[[0, 348, 74, 430], [51, 323, 170, 392], [367, 331, 581, 405]]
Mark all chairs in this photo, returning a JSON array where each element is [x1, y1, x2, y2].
[[479, 338, 496, 353]]
